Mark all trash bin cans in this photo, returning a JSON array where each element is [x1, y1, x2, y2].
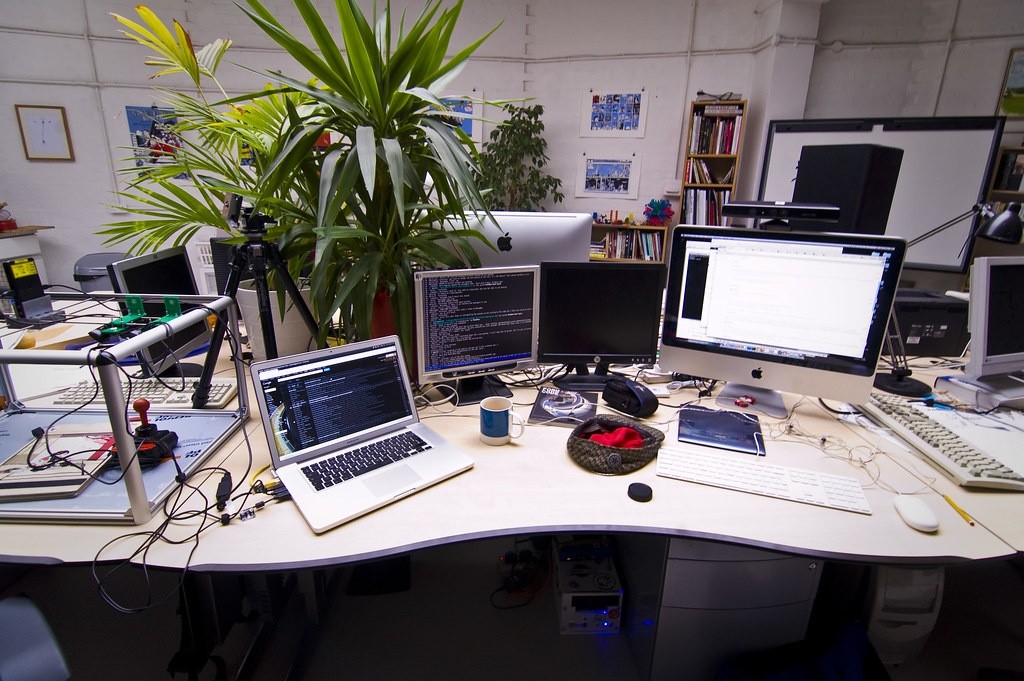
[[74, 253, 133, 293]]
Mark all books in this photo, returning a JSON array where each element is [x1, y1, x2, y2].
[[679, 105, 743, 154], [0, 421, 142, 504], [982, 202, 1024, 224], [527, 386, 601, 428], [589, 230, 661, 261], [682, 187, 729, 228], [983, 152, 1024, 192], [685, 157, 736, 186]]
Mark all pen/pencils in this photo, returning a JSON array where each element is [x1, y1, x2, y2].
[[943, 495, 974, 526]]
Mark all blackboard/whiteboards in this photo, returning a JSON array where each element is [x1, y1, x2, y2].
[[752, 115, 1008, 275]]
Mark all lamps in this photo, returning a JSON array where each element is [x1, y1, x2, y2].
[[872, 202, 1024, 399]]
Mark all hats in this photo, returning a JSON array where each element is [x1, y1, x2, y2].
[[566, 413, 665, 476]]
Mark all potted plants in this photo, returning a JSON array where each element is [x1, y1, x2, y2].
[[93, 0, 537, 383]]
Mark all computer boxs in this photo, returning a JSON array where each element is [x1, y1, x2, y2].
[[820, 563, 947, 665]]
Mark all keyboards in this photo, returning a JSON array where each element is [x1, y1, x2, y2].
[[53, 378, 238, 408], [655, 446, 872, 515], [849, 392, 1024, 492]]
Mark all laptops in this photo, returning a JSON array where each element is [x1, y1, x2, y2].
[[250, 335, 476, 533]]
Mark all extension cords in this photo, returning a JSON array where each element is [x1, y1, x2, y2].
[[497, 560, 527, 574]]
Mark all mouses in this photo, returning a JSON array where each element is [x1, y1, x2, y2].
[[894, 494, 939, 532]]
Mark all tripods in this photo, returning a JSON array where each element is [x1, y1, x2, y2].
[[191, 192, 331, 408]]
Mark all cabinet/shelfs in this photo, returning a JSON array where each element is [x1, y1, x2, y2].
[[961, 146, 1024, 292], [677, 99, 748, 227], [589, 224, 668, 263]]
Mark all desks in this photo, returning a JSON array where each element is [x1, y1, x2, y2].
[[0, 292, 1024, 681]]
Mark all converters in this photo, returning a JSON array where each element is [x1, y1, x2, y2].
[[503, 571, 535, 593]]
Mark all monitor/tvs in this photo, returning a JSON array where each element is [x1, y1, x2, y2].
[[409, 205, 907, 419], [955, 256, 1024, 397], [106, 245, 213, 378]]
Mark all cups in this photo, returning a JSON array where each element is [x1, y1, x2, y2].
[[479, 395, 525, 446]]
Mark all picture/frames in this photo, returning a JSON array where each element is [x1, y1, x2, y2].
[[995, 47, 1024, 122], [15, 104, 75, 161]]
